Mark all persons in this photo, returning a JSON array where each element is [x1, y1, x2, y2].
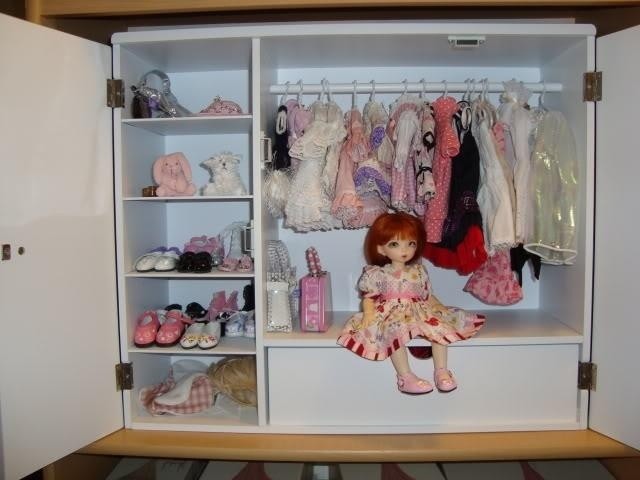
[[356, 212, 457, 393]]
